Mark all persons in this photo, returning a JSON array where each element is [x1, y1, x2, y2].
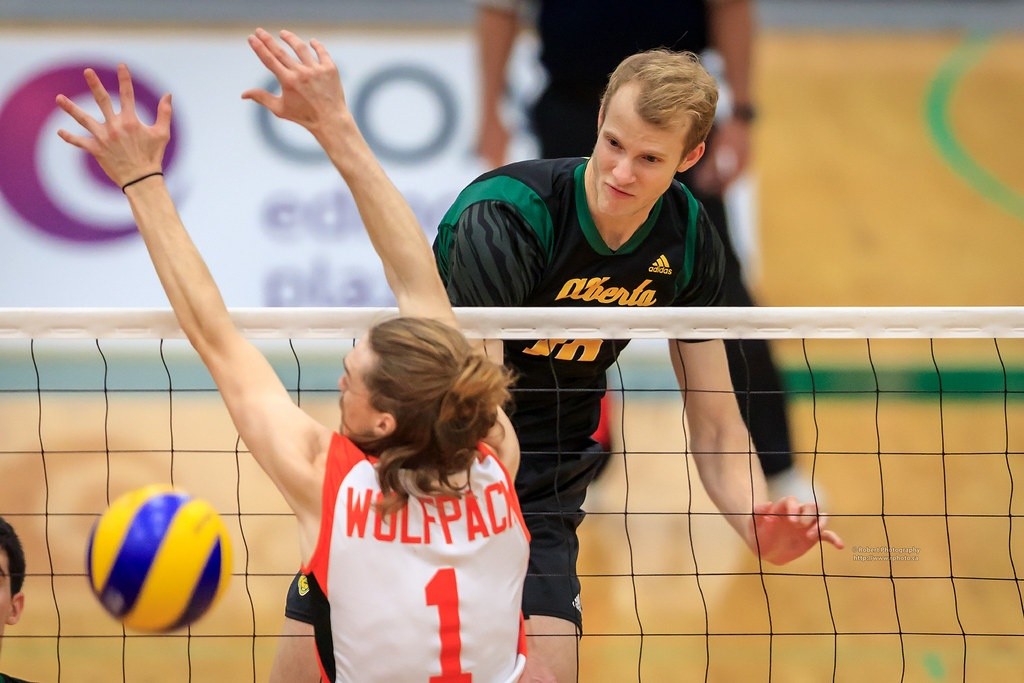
[[460, 0, 823, 524], [0, 515, 38, 683], [267, 48, 846, 681], [48, 28, 540, 683]]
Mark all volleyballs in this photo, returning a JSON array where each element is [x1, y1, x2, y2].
[[89, 485, 230, 637]]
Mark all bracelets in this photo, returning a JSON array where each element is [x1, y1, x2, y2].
[[114, 171, 168, 197], [730, 102, 757, 122]]
